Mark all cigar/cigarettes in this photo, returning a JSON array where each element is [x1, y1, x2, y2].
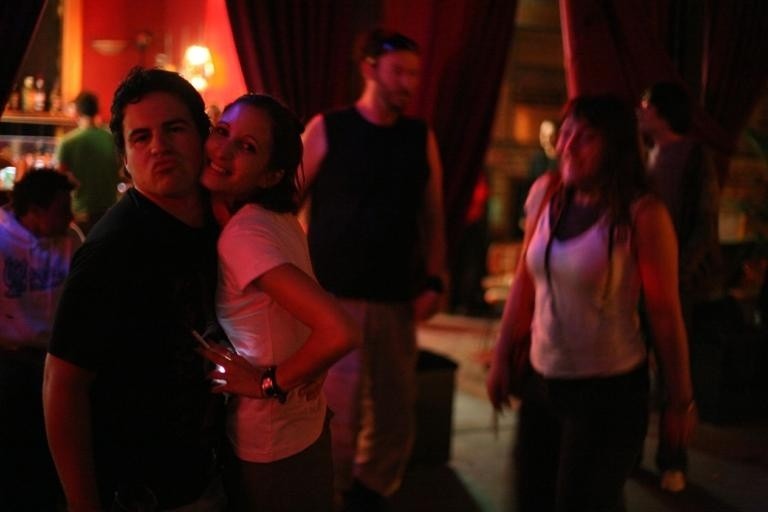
[[192, 330, 214, 352]]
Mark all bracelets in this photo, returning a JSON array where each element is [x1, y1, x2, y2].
[[674, 400, 695, 414], [426, 277, 444, 292]]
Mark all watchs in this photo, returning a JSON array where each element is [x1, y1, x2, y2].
[[261, 366, 286, 404]]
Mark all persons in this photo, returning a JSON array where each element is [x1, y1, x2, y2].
[[295, 29, 450, 512], [625, 80, 727, 493], [522, 118, 562, 248], [0, 169, 86, 351], [58, 95, 123, 236], [199, 94, 364, 512], [42, 70, 328, 512], [487, 93, 693, 512]]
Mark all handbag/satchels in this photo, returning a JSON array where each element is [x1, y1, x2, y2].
[[505, 332, 533, 398]]
